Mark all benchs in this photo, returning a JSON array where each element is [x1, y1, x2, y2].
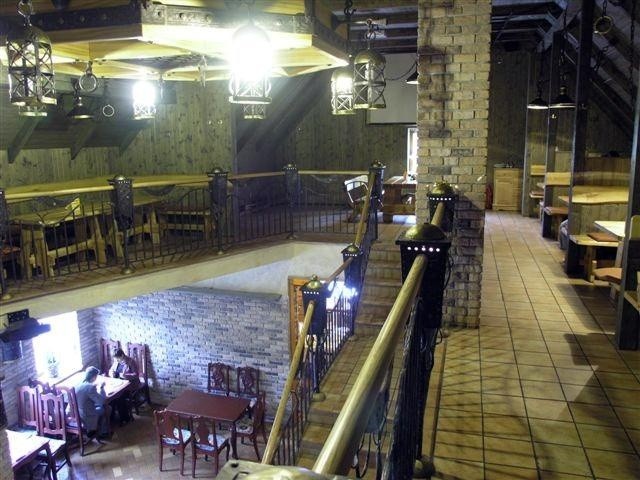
[[344, 174, 381, 223], [529, 162, 640, 358], [7, 195, 209, 282]]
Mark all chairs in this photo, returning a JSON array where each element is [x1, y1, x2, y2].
[[10, 337, 152, 479], [152, 363, 268, 477]]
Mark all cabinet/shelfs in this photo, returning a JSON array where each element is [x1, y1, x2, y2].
[[493, 167, 524, 212]]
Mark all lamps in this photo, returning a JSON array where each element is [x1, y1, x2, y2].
[[66, 93, 94, 120], [5, 0, 63, 117], [332, 1, 389, 117], [526, 2, 577, 110]]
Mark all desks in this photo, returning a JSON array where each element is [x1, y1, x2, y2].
[[1, 172, 223, 205], [382, 176, 419, 224], [6, 430, 56, 480]]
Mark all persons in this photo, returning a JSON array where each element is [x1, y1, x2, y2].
[[71, 365, 115, 441], [107, 347, 141, 428]]
